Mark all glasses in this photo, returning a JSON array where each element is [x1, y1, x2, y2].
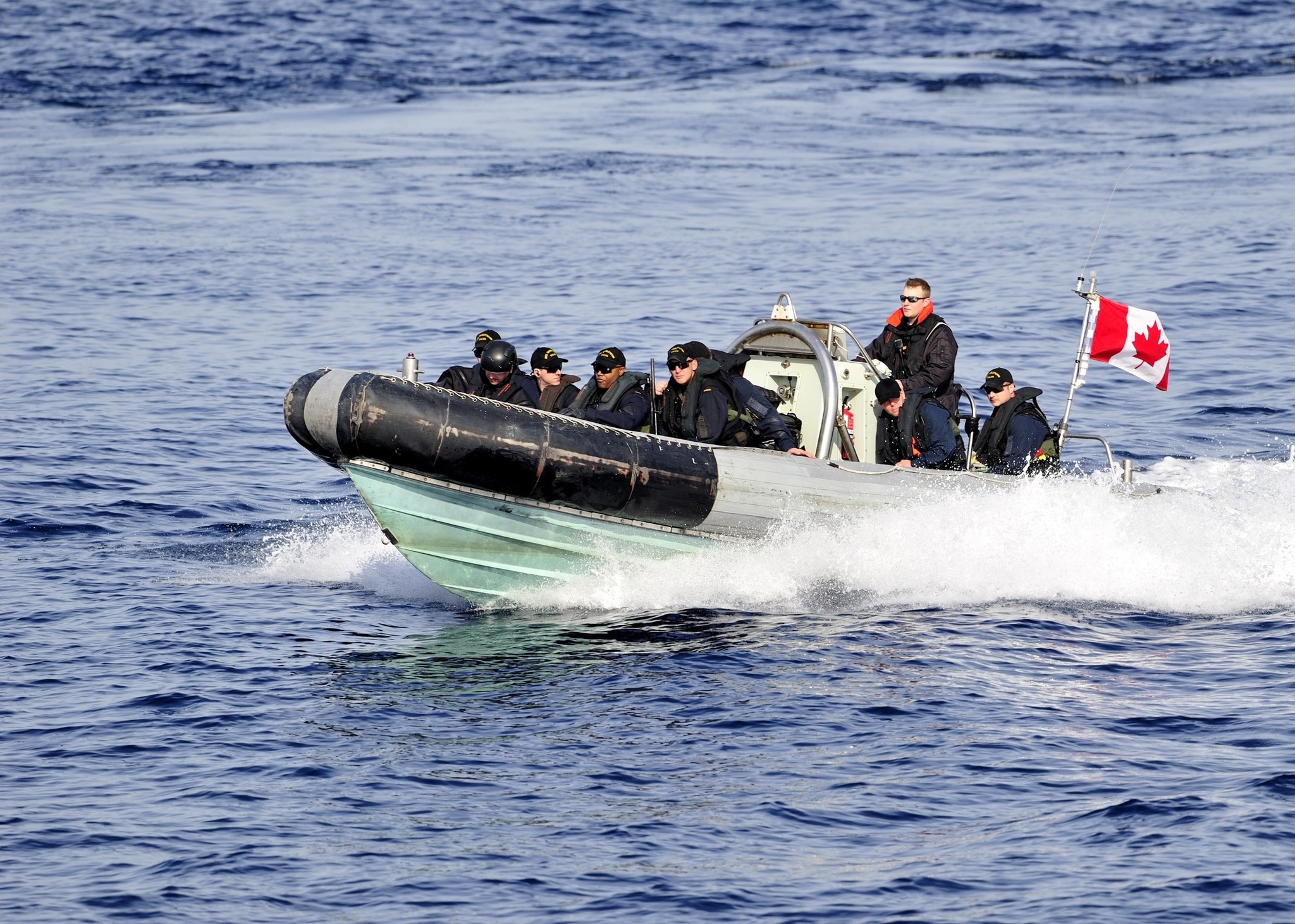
[[900, 295, 929, 303], [984, 383, 1012, 394], [593, 363, 623, 374], [666, 360, 693, 370], [540, 363, 562, 373]]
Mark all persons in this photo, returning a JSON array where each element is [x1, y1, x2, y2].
[[654, 342, 816, 458], [851, 278, 961, 415], [557, 347, 653, 432], [975, 367, 1059, 475], [873, 379, 968, 470], [528, 346, 581, 416], [424, 330, 540, 408]]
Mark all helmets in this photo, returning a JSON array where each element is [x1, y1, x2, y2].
[[480, 340, 517, 373]]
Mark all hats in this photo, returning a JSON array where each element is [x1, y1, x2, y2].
[[980, 367, 1013, 389], [475, 330, 501, 357], [875, 378, 901, 405], [531, 347, 568, 370], [591, 347, 626, 368], [667, 344, 694, 364]]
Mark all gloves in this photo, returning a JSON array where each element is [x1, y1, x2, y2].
[[965, 417, 981, 436], [558, 407, 585, 418]]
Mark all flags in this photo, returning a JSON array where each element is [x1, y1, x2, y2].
[[1090, 294, 1170, 392]]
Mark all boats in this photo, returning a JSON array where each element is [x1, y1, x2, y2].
[[282, 290, 1135, 620]]
